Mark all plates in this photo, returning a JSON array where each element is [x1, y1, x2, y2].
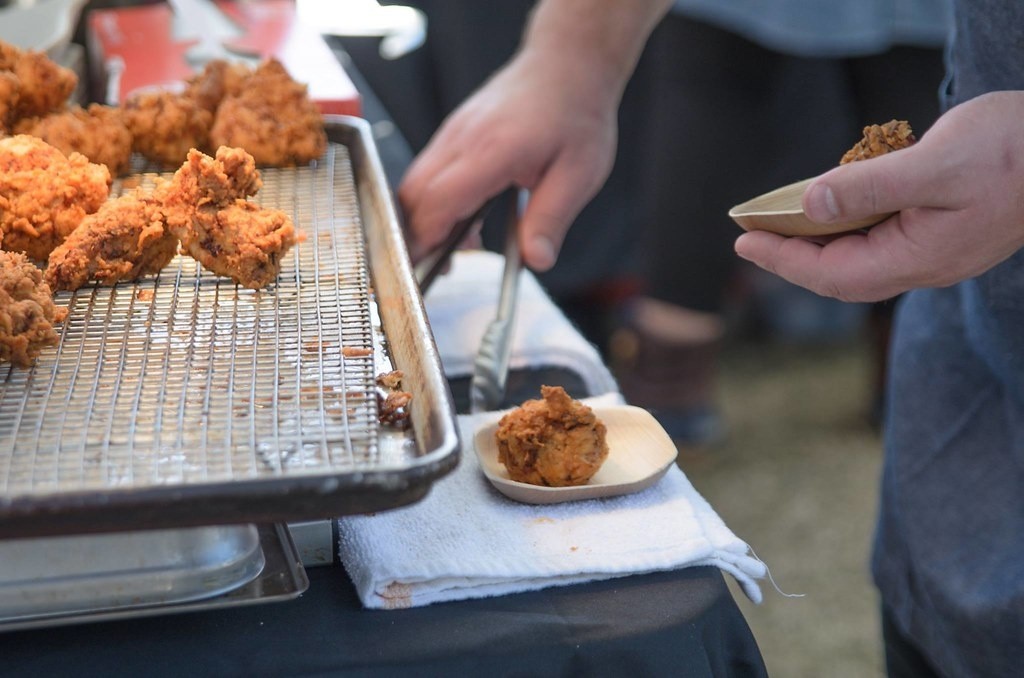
[[729, 171, 897, 238], [472, 403, 680, 507]]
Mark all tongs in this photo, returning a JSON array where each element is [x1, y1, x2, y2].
[[410, 185, 527, 411]]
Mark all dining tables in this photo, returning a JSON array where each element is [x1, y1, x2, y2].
[[2, 1, 766, 678]]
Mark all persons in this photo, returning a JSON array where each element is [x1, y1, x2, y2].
[[395, 0, 1024, 678]]
